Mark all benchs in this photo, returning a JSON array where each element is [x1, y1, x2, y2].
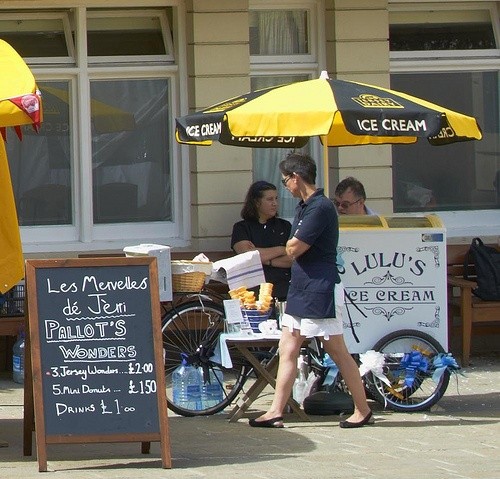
[[447, 243, 500, 368]]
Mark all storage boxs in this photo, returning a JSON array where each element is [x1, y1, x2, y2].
[[1, 287, 26, 318], [124, 244, 174, 303]]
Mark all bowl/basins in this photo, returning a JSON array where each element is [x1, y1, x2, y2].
[[241, 309, 270, 334]]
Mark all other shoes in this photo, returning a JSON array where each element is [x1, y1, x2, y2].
[[248, 416, 285, 428], [340, 410, 373, 428]]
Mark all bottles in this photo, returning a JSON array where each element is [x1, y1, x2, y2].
[[172, 354, 202, 414], [293, 346, 308, 409], [197, 365, 223, 413], [12, 331, 25, 384]]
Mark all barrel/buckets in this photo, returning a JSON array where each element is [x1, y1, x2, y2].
[[275, 298, 287, 329]]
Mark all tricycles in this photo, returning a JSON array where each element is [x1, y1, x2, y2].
[[162, 272, 451, 417]]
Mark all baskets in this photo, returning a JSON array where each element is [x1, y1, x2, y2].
[[171, 260, 212, 293]]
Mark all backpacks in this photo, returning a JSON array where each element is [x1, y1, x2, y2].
[[463, 237, 500, 302]]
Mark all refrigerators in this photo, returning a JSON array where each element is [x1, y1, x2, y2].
[[335, 212, 449, 353]]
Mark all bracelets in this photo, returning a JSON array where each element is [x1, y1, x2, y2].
[[270, 260, 272, 267]]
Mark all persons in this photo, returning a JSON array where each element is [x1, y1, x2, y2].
[[249, 152, 374, 428], [335, 177, 376, 215], [230, 180, 292, 351]]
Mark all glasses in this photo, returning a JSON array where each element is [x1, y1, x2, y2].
[[334, 199, 360, 209], [281, 174, 292, 186]]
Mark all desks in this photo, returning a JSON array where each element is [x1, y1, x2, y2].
[[221, 333, 314, 427]]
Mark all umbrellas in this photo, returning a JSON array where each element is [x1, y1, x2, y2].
[[0, 39, 43, 141], [175, 71, 483, 199]]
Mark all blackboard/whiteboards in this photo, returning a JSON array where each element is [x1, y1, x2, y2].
[[24, 256, 170, 442]]
